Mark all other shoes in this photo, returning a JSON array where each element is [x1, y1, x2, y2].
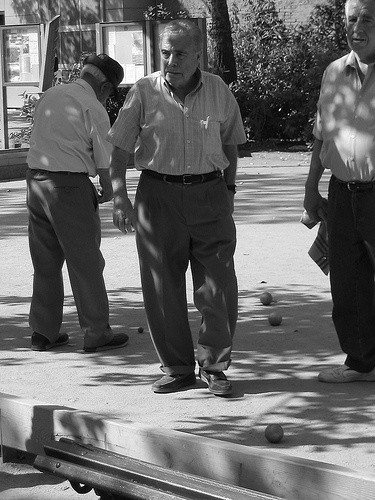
[[152, 371, 196, 394], [318, 366, 375, 383], [31, 332, 69, 351], [199, 368, 232, 396], [83, 333, 129, 352]]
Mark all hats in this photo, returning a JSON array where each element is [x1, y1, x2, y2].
[[82, 53, 124, 93]]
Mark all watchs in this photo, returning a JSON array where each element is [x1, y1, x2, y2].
[[226, 184, 237, 193]]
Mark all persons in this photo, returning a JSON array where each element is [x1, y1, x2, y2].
[[303, 0, 375, 382], [106, 17, 247, 395], [25, 53, 129, 352]]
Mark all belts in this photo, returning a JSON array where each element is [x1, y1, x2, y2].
[[142, 168, 222, 185], [32, 168, 88, 176], [331, 176, 375, 192]]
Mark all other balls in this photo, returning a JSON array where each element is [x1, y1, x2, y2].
[[138, 327, 144, 333], [259, 291, 273, 306], [264, 423, 284, 444], [268, 311, 282, 326]]
